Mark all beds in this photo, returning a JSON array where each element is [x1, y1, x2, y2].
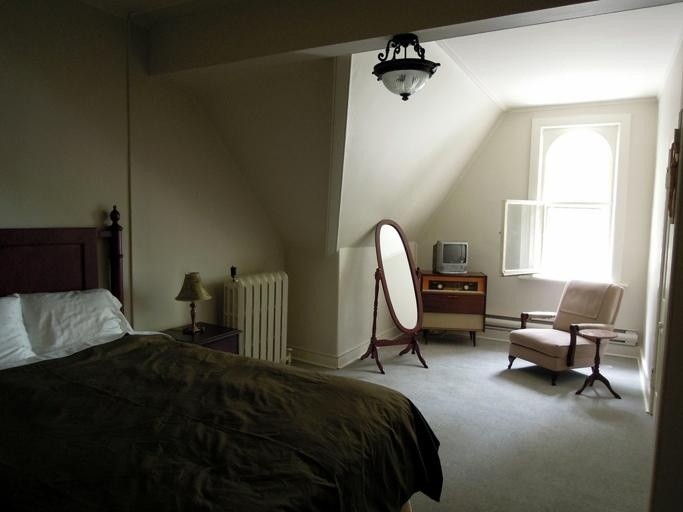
[[0, 204, 421, 510]]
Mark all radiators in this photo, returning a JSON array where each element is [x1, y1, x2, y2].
[[219, 271, 288, 367]]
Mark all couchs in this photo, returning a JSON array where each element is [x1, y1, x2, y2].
[[507, 276, 625, 387]]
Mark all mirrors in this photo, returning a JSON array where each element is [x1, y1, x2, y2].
[[359, 220, 428, 375]]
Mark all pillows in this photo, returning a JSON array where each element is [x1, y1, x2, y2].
[[13, 287, 134, 363], [0, 296, 30, 365]]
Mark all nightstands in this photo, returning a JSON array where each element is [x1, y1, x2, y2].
[[158, 324, 242, 353]]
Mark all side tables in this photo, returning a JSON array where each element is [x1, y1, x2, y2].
[[575, 328, 639, 394]]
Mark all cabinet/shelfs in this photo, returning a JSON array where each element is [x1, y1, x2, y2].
[[417, 267, 488, 296]]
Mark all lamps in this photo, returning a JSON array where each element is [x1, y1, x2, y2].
[[172, 269, 210, 334], [371, 25, 441, 100]]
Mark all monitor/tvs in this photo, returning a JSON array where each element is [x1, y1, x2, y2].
[[433, 240, 469, 275]]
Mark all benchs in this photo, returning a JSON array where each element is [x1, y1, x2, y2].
[[413, 290, 489, 347]]
